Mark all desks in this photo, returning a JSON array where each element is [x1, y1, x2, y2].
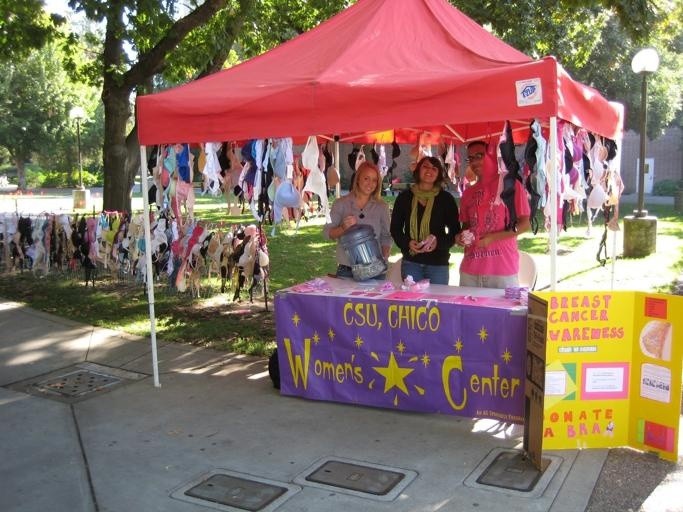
[[274, 275, 529, 424]]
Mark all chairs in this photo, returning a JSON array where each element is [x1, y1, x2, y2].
[[517, 252, 539, 295]]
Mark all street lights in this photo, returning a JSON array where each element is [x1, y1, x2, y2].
[[631, 46, 660, 216], [69, 106, 86, 189]]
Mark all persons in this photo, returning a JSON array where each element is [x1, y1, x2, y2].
[[391, 156, 461, 285], [453, 141, 531, 290], [322, 162, 392, 281]]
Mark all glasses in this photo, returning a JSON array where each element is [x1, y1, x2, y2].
[[465, 153, 482, 163]]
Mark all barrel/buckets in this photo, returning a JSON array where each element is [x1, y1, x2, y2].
[[340, 225, 388, 282]]
